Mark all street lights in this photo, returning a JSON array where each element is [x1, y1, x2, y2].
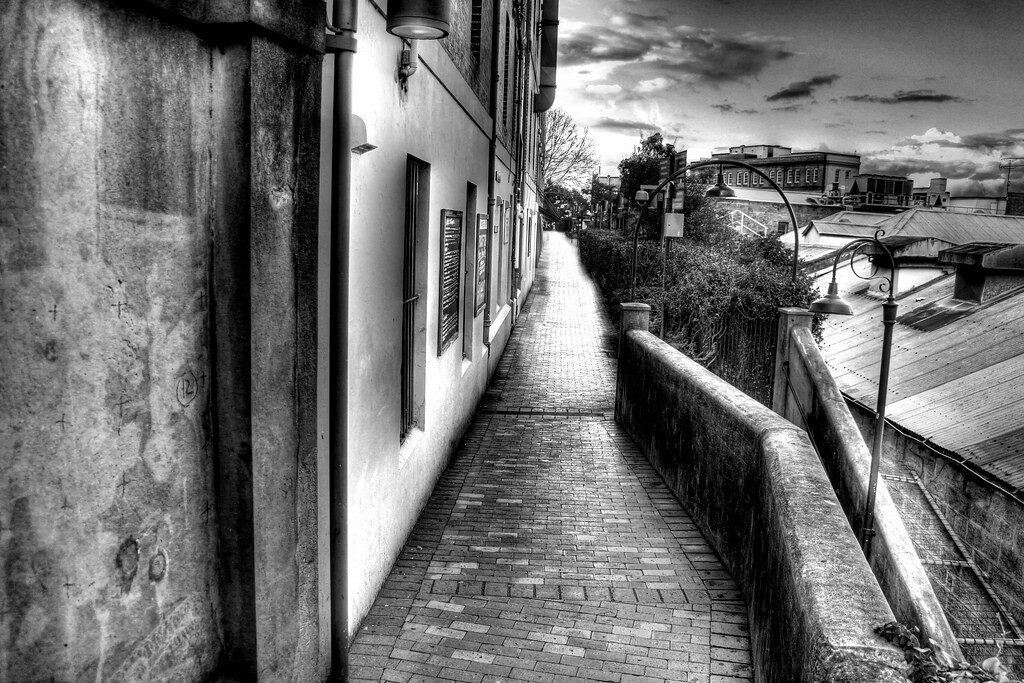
[[806, 230, 900, 558]]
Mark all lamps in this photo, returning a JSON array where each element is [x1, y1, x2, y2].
[[386, 0, 450, 39]]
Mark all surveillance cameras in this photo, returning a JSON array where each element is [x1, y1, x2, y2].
[[636, 191, 649, 205]]
